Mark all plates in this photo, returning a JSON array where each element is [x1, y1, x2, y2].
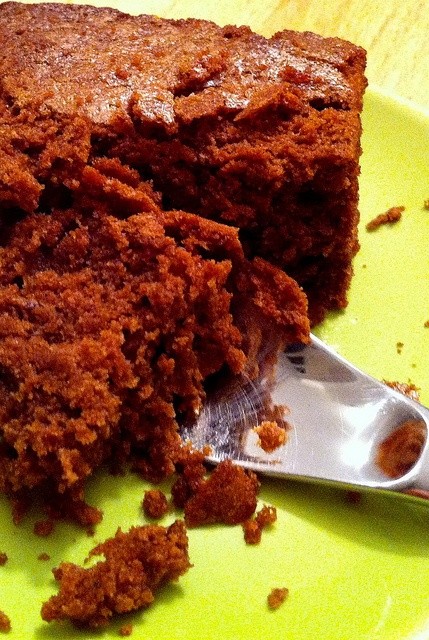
[[1, 87, 429, 635]]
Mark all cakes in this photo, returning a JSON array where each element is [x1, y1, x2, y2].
[[2, 0, 367, 628]]
